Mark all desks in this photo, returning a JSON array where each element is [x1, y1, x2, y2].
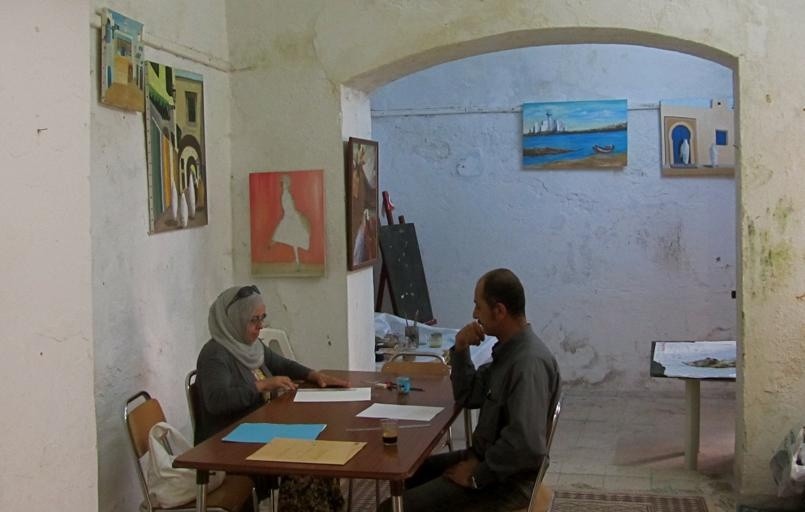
[[649, 339, 737, 471], [376, 311, 497, 368], [173, 368, 474, 512]]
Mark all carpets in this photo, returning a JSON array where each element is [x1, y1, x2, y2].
[[554, 491, 708, 512]]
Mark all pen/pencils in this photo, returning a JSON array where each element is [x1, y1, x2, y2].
[[409, 387, 423, 392]]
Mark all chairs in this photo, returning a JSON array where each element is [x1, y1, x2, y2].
[[184, 369, 275, 512], [521, 390, 566, 512], [121, 391, 258, 512], [381, 351, 457, 454]]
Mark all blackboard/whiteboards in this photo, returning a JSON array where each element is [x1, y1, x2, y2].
[[375, 223, 434, 328]]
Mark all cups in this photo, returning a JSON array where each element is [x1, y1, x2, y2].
[[431, 333, 442, 348], [405, 326, 420, 347], [381, 419, 399, 446], [397, 377, 411, 394]]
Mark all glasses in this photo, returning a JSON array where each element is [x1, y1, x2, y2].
[[224, 284, 262, 314]]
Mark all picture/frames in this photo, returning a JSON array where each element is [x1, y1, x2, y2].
[[347, 136, 380, 271], [248, 171, 326, 279]]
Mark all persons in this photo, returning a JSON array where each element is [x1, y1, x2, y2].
[[377, 268, 559, 512], [351, 143, 377, 266], [271, 174, 311, 265], [192, 285, 344, 512]]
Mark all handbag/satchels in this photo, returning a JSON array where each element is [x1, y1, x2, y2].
[[144, 421, 227, 508]]
